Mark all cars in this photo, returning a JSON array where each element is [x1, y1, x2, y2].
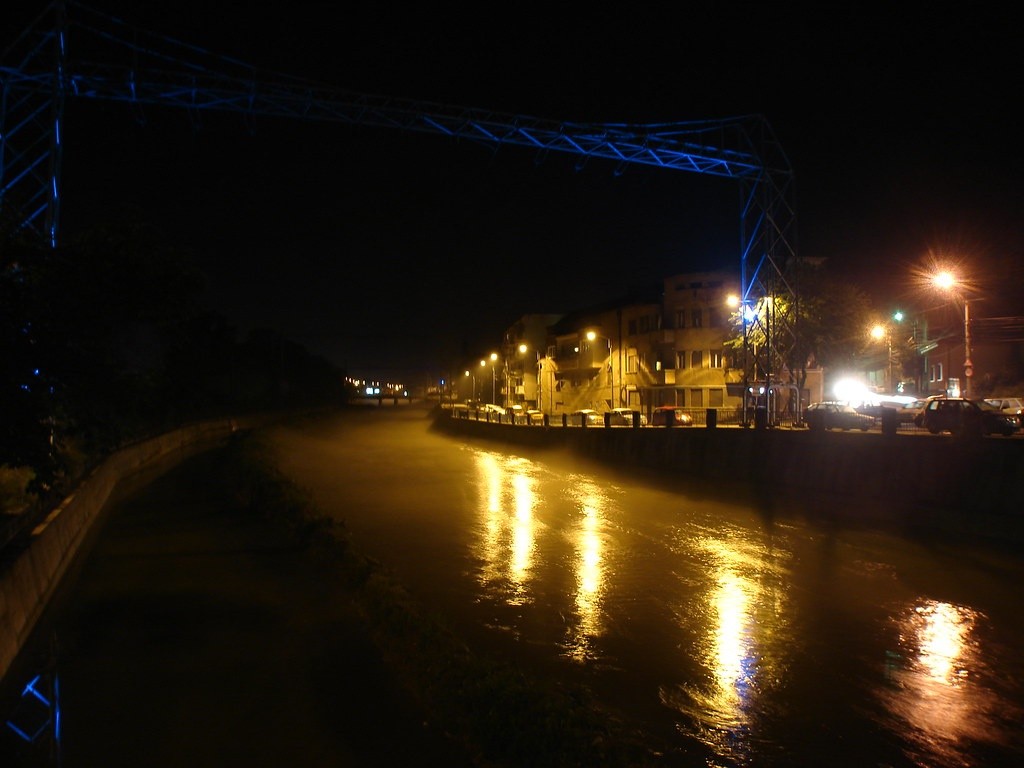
[[572, 409, 604, 426], [803, 399, 875, 432], [610, 409, 648, 426], [988, 397, 1024, 418], [892, 395, 937, 422], [653, 407, 692, 426], [507, 406, 545, 426]]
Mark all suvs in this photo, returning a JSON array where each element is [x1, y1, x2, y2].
[[923, 398, 1022, 438]]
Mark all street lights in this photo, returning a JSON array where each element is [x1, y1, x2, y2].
[[934, 272, 975, 397], [519, 345, 542, 413], [481, 360, 495, 405], [465, 370, 476, 400], [587, 331, 615, 409], [874, 327, 894, 395]]
